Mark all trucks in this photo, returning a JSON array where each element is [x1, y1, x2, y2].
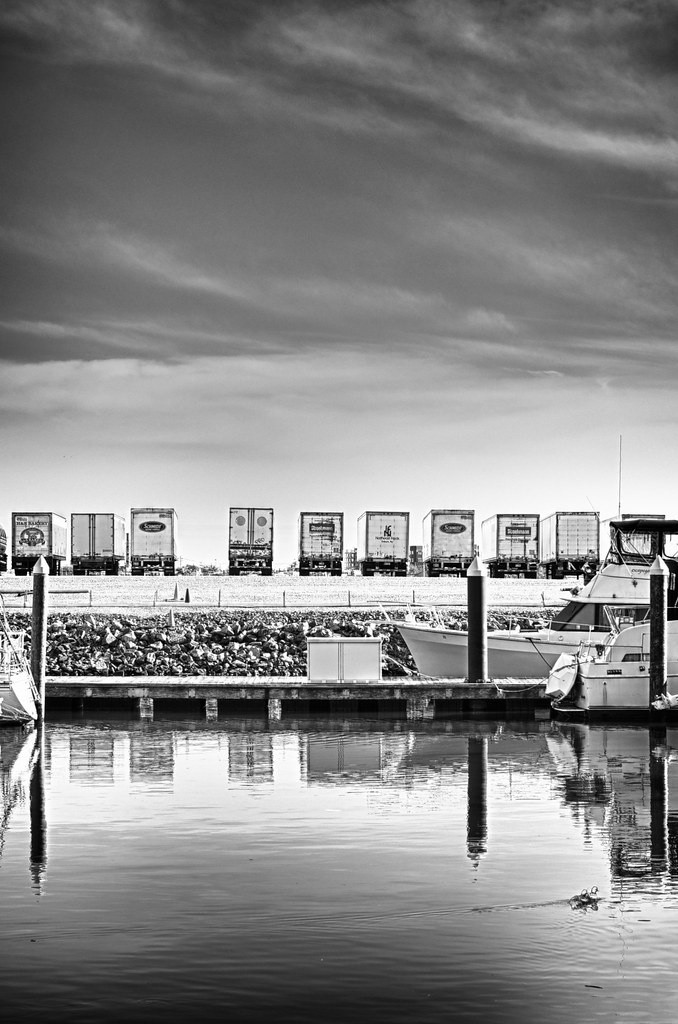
[[71, 512, 127, 577], [130, 506, 179, 576], [296, 509, 600, 579], [227, 506, 275, 575], [10, 511, 67, 575]]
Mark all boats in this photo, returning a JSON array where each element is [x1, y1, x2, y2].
[[0, 609, 41, 723], [372, 518, 678, 713]]
